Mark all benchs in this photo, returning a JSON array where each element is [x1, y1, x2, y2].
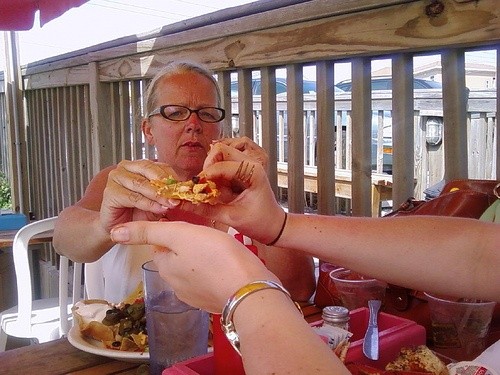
[[276, 162, 417, 218]]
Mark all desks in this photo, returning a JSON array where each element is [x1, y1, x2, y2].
[[0, 267, 500, 375], [0, 219, 53, 249]]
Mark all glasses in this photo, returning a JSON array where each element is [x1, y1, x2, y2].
[[148, 103, 226, 123]]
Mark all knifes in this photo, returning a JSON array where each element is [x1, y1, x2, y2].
[[363, 299, 381, 361]]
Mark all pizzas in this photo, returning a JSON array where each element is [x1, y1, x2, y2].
[[150, 175, 223, 207]]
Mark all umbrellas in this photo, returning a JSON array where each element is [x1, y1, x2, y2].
[[0, 0, 91, 32]]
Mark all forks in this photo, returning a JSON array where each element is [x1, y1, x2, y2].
[[232, 161, 255, 192]]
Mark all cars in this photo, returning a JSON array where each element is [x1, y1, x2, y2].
[[231, 78, 442, 176]]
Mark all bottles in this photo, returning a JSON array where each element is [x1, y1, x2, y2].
[[321, 306, 349, 332]]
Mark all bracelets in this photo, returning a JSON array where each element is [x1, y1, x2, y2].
[[220, 280, 303, 357], [266, 212, 287, 246]]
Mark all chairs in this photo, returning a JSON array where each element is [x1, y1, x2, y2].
[[0, 215, 105, 356]]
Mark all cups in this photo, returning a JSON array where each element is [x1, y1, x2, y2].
[[141, 260, 208, 375], [330, 268, 386, 312], [212, 312, 246, 375], [424, 291, 496, 363]]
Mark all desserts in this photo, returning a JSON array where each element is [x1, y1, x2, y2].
[[70, 299, 119, 341]]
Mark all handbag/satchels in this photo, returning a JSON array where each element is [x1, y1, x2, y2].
[[385, 181, 500, 220]]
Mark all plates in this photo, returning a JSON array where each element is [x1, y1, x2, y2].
[[69, 299, 212, 360]]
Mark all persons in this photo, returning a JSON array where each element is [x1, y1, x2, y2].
[[53, 61, 268, 306], [110, 144, 500, 375]]
